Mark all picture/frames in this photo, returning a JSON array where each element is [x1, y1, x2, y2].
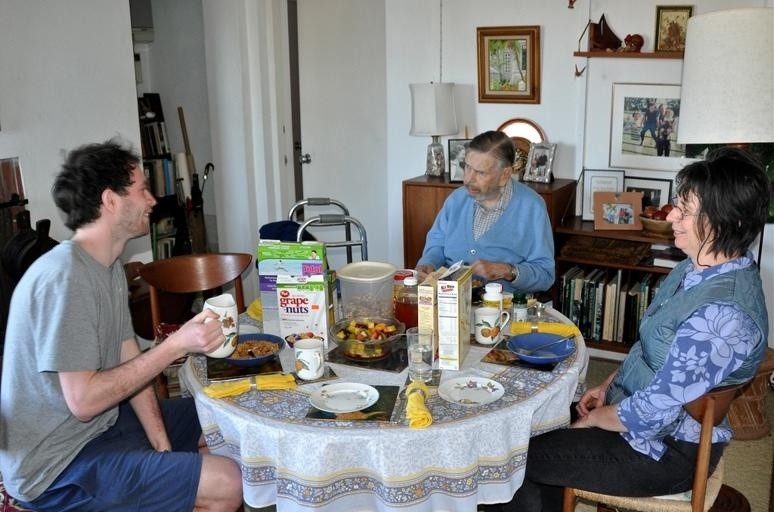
[[654, 5, 693, 51], [448, 139, 472, 183], [624, 176, 673, 212], [609, 82, 709, 172], [581, 169, 625, 222], [523, 141, 557, 183], [477, 26, 540, 104], [594, 192, 642, 230]]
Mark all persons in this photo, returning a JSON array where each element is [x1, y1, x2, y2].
[[413, 130, 557, 293], [530, 153, 539, 175], [538, 151, 547, 176], [501, 155, 772, 511], [0, 136, 244, 512], [624, 101, 676, 158], [451, 144, 466, 179]]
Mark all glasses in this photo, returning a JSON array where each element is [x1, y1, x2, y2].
[[672, 196, 707, 218]]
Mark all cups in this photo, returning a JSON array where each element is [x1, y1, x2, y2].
[[406, 327, 433, 384], [293, 339, 323, 381], [202, 293, 239, 359], [474, 307, 511, 345]]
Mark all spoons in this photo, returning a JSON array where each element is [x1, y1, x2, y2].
[[515, 332, 575, 356]]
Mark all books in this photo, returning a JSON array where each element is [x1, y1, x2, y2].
[[142, 122, 178, 260], [653, 246, 688, 269], [559, 265, 668, 343]]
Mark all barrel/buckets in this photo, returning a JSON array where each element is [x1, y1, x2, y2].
[[336, 262, 395, 319]]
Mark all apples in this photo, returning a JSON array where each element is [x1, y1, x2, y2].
[[644, 205, 673, 220]]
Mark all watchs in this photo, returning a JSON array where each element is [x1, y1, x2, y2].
[[503, 263, 518, 282]]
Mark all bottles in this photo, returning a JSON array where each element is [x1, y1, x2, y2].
[[482, 282, 528, 320], [396, 277, 417, 328]]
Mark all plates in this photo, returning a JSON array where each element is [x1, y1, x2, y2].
[[505, 333, 576, 364], [308, 383, 379, 415], [437, 376, 505, 407], [225, 333, 284, 365]]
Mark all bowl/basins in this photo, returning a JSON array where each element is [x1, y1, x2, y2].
[[328, 316, 406, 362], [638, 213, 673, 234]]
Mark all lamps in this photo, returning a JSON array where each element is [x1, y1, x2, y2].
[[674, 6, 774, 145], [408, 82, 460, 177]]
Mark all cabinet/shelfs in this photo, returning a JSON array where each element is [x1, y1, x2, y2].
[[402, 172, 576, 270], [554, 217, 687, 360], [138, 93, 192, 260]]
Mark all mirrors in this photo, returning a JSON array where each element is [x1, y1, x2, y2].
[[496, 118, 545, 145]]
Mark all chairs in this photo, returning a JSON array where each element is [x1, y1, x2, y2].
[[564, 383, 752, 512], [138, 253, 252, 399], [0, 480, 33, 512]]
